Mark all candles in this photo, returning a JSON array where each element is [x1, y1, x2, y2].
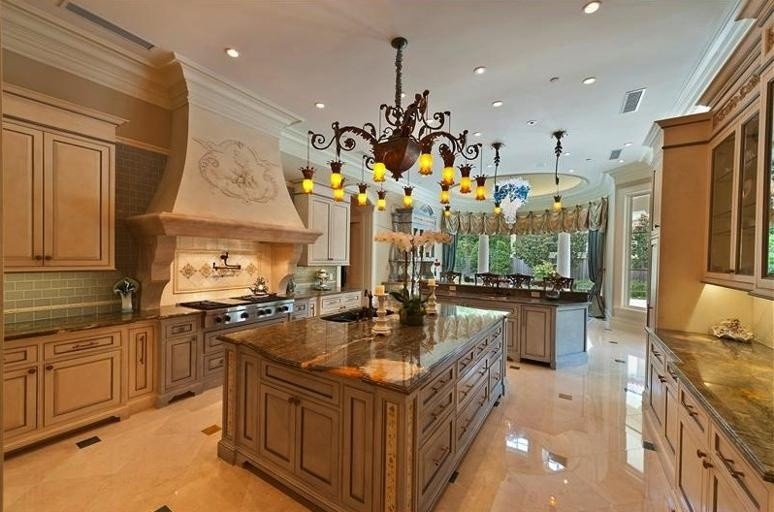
[[374, 286, 385, 296], [426, 278, 436, 285]]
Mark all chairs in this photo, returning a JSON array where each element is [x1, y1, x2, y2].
[[440, 268, 605, 319]]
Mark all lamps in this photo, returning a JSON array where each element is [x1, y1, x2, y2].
[[494, 176, 530, 229], [551, 129, 569, 210], [489, 140, 503, 215], [298, 36, 488, 216]]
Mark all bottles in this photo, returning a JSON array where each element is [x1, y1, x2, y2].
[[365, 293, 374, 319], [362, 289, 369, 309]]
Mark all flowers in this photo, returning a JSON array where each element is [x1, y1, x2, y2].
[[373, 230, 454, 311]]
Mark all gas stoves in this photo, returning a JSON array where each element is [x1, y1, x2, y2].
[[178, 294, 294, 329]]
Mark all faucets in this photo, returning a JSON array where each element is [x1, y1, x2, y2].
[[363, 289, 376, 317]]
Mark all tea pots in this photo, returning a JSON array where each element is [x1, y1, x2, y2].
[[247, 276, 268, 295]]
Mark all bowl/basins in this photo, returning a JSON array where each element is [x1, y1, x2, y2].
[[711, 325, 754, 342]]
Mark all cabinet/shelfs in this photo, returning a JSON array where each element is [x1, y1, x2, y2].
[[0, 324, 126, 452], [1, 82, 130, 271], [290, 178, 353, 268], [294, 287, 364, 321], [238, 349, 376, 512], [123, 320, 155, 417], [204, 317, 291, 386], [697, 21, 774, 298], [156, 313, 202, 409], [646, 343, 772, 511], [436, 295, 549, 366], [419, 319, 506, 512]]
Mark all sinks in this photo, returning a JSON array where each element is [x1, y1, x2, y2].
[[320, 307, 394, 323]]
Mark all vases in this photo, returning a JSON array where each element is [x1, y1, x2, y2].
[[398, 308, 427, 326]]
